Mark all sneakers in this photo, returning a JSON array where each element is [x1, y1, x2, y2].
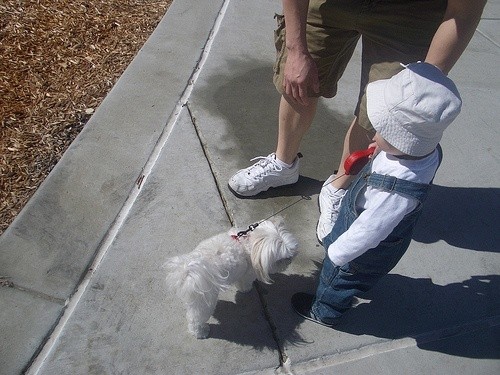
[[316, 169, 354, 248], [227, 151, 303, 200]]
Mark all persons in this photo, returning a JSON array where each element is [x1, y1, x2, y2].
[[291, 62, 463, 328], [227, 0, 489, 249]]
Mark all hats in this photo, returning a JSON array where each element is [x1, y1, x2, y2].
[[365, 60, 463, 157]]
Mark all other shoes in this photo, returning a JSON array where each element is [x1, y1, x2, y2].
[[290, 292, 341, 328]]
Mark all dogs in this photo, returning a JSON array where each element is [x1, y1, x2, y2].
[[163, 213, 299, 339]]
